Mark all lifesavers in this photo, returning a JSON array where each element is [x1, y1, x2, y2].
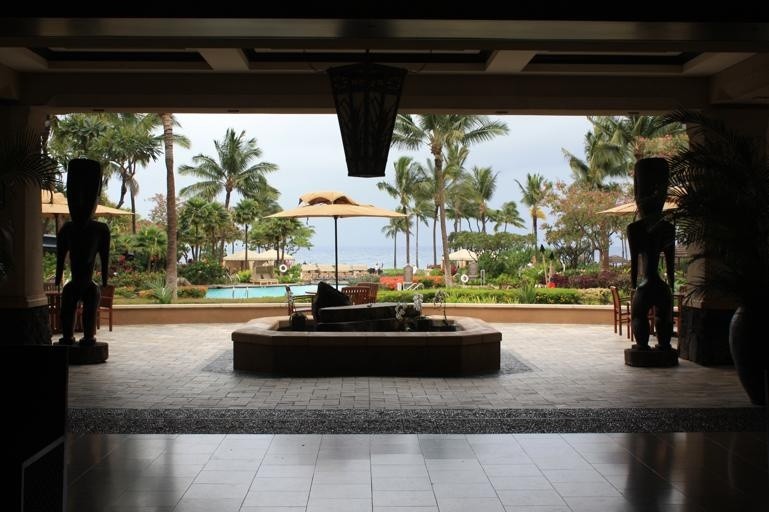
[[279, 264, 287, 272], [461, 274, 469, 283]]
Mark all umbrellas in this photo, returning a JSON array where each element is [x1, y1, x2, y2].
[[262, 190, 408, 290], [39, 189, 137, 290], [225, 248, 260, 271], [253, 248, 293, 278], [595, 201, 683, 217], [442, 248, 480, 268]]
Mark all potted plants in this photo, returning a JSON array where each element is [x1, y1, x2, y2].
[[649, 102, 769, 405]]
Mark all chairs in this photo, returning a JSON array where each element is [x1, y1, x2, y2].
[[43, 279, 114, 335], [283, 282, 379, 318], [609, 283, 685, 341]]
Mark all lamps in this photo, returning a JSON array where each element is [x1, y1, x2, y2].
[[294, 42, 435, 180]]
